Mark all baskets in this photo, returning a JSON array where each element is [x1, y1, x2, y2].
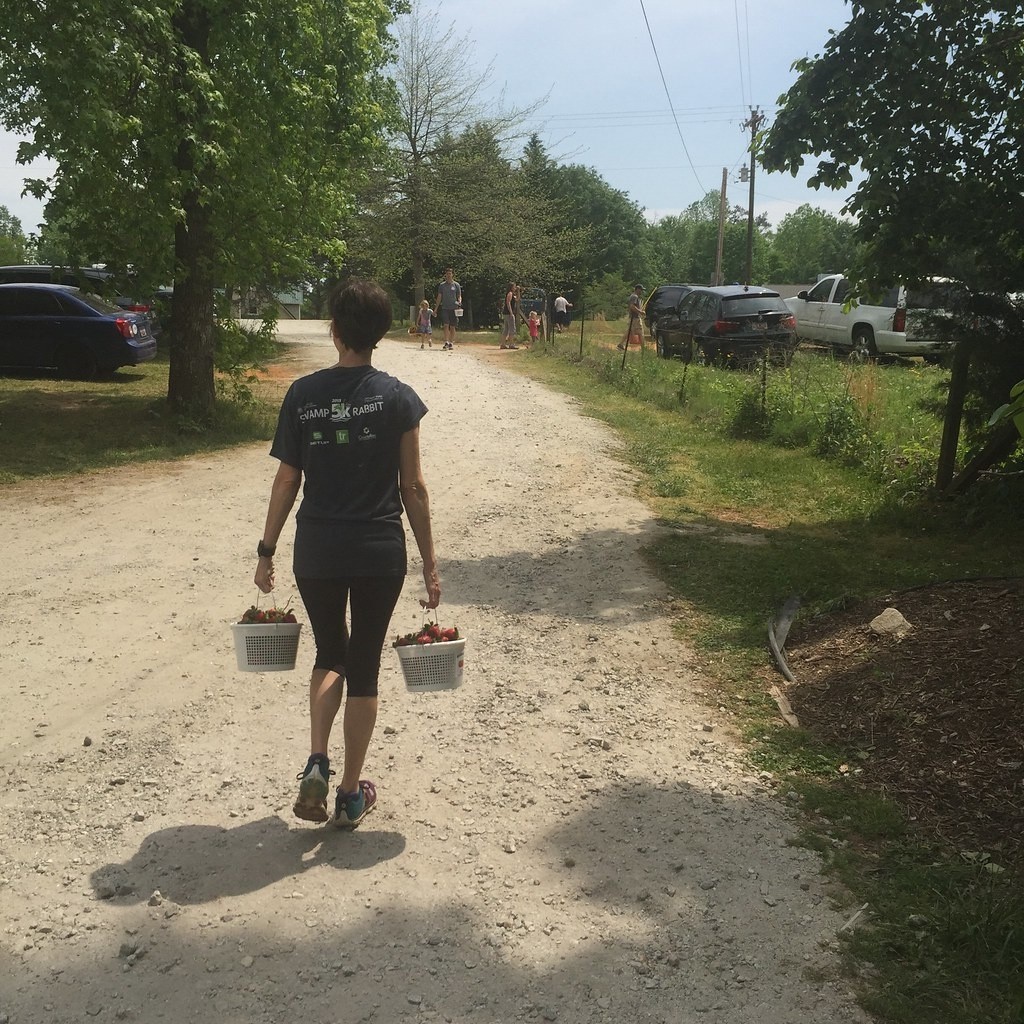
[[454, 303, 464, 316], [396, 606, 467, 693], [230, 589, 304, 672]]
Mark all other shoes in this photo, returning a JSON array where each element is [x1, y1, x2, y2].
[[616, 344, 625, 350], [449, 343, 453, 350], [500, 344, 509, 349], [509, 344, 519, 349], [421, 344, 424, 349], [429, 341, 433, 347], [443, 343, 449, 349]]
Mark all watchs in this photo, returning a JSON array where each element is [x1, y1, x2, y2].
[[257, 539, 277, 557]]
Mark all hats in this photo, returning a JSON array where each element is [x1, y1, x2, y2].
[[636, 284, 646, 290]]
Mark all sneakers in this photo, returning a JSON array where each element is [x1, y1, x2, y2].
[[293, 753, 336, 821], [335, 780, 377, 827]]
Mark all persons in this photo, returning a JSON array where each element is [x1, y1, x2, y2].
[[254, 282, 440, 831], [552, 295, 573, 333], [500, 282, 541, 350], [433, 269, 462, 350], [416, 300, 437, 349], [617, 284, 646, 350]]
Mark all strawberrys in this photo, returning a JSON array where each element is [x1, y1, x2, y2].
[[242, 605, 296, 624], [396, 623, 458, 644]]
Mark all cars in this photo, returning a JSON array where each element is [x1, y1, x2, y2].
[[0, 281, 158, 380]]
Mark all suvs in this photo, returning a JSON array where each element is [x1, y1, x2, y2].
[[0, 264, 175, 339], [783, 270, 975, 364], [642, 282, 711, 342], [655, 283, 799, 372]]
